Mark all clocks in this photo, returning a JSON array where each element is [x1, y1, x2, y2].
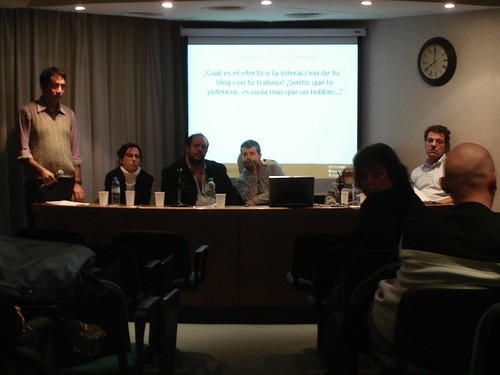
[[417, 37, 456, 87]]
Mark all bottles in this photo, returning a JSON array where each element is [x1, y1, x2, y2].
[[352, 179, 360, 206], [206, 178, 216, 208], [111, 176, 121, 207]]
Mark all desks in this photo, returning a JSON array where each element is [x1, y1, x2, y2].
[[34, 204, 456, 324]]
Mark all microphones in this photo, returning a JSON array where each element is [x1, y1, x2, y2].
[[39, 169, 65, 188]]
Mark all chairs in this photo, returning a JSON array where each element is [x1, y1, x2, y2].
[[0, 229, 210, 375], [286, 233, 500, 375]]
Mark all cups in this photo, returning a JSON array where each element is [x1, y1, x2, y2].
[[215, 193, 226, 208], [98, 190, 109, 205], [341, 191, 349, 204], [154, 191, 165, 207], [125, 190, 135, 206]]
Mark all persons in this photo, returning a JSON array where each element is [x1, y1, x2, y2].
[[161, 133, 246, 220], [403, 124, 462, 205], [235, 140, 286, 208], [15, 66, 87, 232], [352, 143, 500, 374], [1, 287, 26, 339], [301, 143, 436, 375], [324, 170, 356, 204], [104, 142, 154, 204]]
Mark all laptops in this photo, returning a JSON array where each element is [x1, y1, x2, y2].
[[268, 174, 314, 206]]
